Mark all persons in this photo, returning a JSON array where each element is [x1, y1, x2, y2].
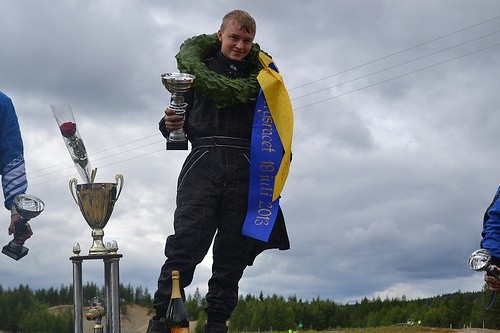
[[143, 8, 295, 333], [0, 90, 34, 239], [480, 180, 500, 293]]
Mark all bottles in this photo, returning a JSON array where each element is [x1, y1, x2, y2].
[[165, 271, 189, 333]]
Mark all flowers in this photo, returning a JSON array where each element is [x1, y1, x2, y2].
[[60, 122, 98, 183]]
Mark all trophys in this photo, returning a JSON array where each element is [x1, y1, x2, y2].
[[468, 248, 498, 286], [4, 190, 44, 264], [67, 167, 125, 257], [158, 69, 195, 151]]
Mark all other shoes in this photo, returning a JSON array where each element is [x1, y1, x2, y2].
[[147, 319, 167, 333]]
